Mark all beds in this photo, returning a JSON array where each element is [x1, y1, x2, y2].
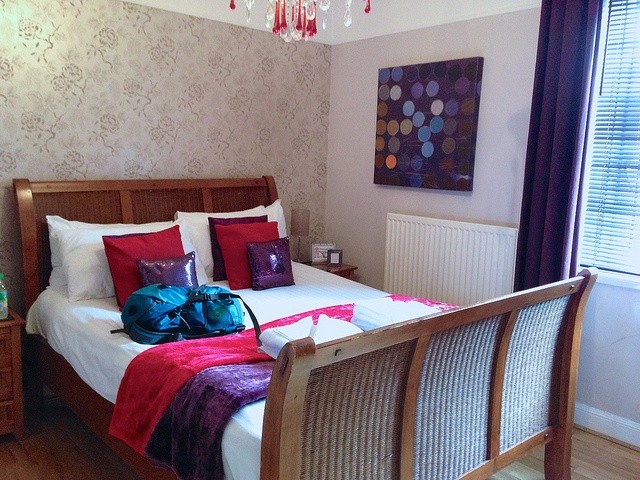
[[12, 173, 599, 479]]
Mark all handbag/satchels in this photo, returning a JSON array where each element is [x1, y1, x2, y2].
[[111, 282, 267, 345]]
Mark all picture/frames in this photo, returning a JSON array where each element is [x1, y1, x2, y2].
[[311, 243, 336, 264]]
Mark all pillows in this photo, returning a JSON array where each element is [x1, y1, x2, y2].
[[214, 222, 280, 290], [208, 215, 268, 282], [101, 225, 186, 313], [246, 236, 295, 290], [44, 215, 211, 289], [136, 250, 199, 288], [174, 199, 288, 286], [56, 221, 207, 302]]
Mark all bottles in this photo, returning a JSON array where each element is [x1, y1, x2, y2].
[[0, 274, 9, 319]]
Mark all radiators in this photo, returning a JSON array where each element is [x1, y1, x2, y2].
[[383, 213, 519, 307]]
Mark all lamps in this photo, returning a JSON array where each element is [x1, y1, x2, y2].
[[226, 0, 372, 46], [290, 207, 310, 262]]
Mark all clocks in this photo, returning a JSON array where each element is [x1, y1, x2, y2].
[[327, 249, 343, 267]]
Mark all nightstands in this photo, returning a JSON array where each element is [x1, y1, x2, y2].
[[296, 259, 357, 280], [0, 307, 27, 440]]
